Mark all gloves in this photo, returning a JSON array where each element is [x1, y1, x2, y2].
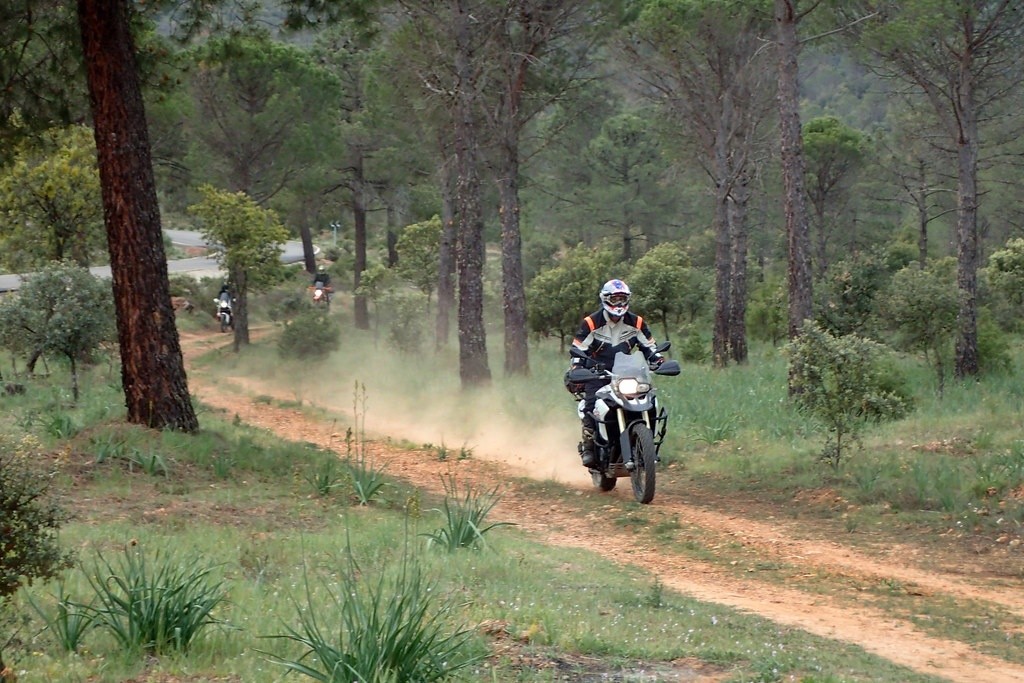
[[656, 357, 664, 367]]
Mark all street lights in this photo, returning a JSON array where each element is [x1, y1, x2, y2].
[[330, 220, 341, 247]]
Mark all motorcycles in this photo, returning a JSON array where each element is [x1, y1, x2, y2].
[[564, 341, 681, 504], [213, 292, 236, 333], [306, 281, 335, 313]]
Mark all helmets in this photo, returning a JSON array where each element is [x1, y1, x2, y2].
[[223, 275, 230, 285], [599, 279, 632, 316], [318, 264, 326, 274]]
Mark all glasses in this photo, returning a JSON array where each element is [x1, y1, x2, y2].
[[600, 294, 631, 306]]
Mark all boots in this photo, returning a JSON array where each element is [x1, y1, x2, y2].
[[581, 426, 594, 466]]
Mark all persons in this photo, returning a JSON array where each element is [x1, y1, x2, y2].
[[313, 266, 332, 305], [214, 282, 235, 321], [570, 279, 664, 467]]
